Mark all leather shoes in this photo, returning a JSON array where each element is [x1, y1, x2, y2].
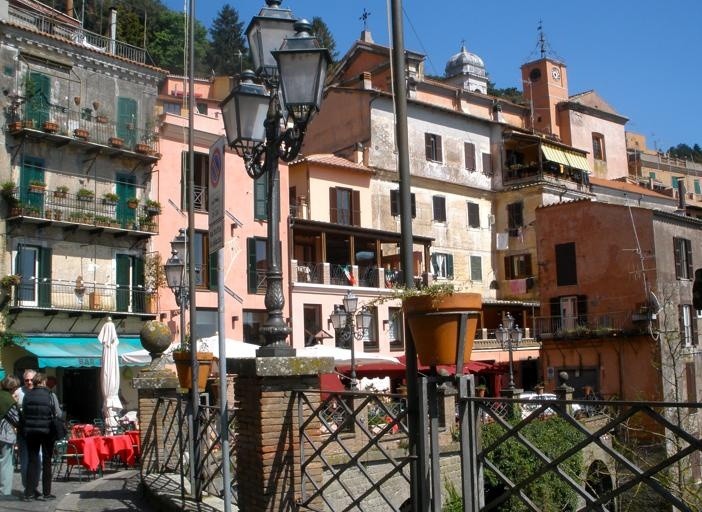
[[24, 493, 35, 499], [43, 494, 56, 499]]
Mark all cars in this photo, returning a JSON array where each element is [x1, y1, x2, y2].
[[508, 394, 583, 420]]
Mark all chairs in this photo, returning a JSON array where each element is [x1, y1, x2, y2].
[[51, 418, 139, 485]]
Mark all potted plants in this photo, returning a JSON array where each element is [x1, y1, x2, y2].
[[361, 279, 483, 364], [55, 185, 70, 198], [143, 200, 161, 213], [169, 333, 213, 390], [76, 188, 95, 202], [127, 197, 139, 208], [101, 193, 120, 205], [28, 179, 48, 194]]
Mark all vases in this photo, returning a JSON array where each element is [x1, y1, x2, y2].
[[43, 122, 57, 132], [108, 137, 125, 148], [135, 143, 149, 153], [74, 129, 89, 139], [7, 121, 22, 131]]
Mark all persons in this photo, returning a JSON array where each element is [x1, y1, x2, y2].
[[0, 369, 62, 502]]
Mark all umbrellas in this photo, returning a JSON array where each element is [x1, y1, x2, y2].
[[296, 341, 400, 365], [97, 317, 124, 437], [121, 331, 262, 364]]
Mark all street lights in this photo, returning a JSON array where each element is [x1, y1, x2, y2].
[[494, 312, 526, 389], [329, 294, 375, 379], [220, 0, 344, 358]]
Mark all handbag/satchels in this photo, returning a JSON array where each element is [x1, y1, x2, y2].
[[50, 420, 67, 440]]
